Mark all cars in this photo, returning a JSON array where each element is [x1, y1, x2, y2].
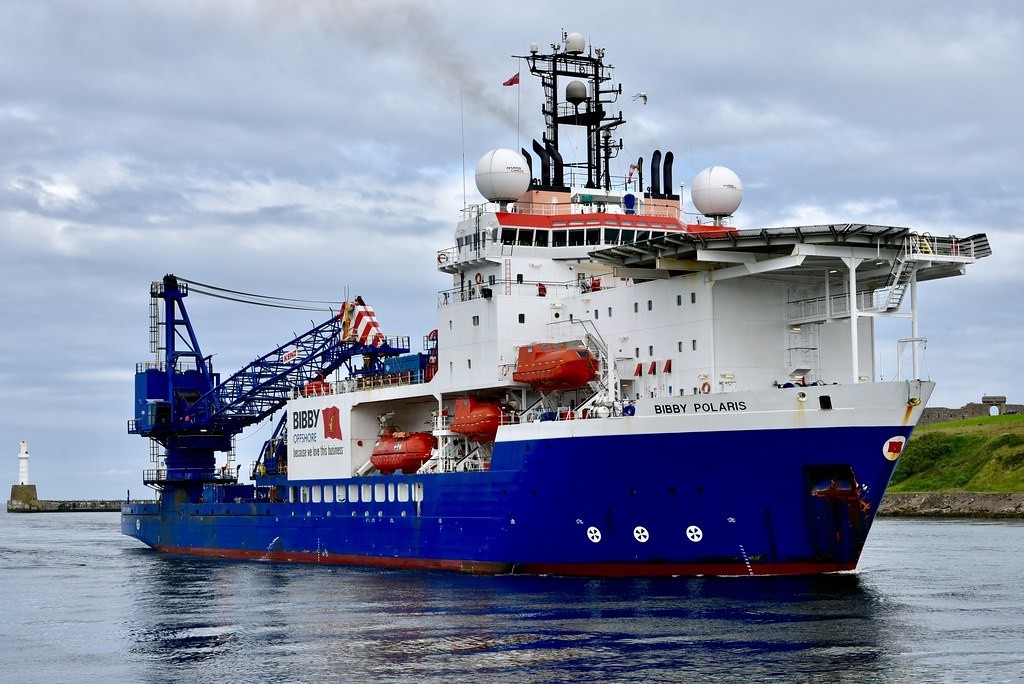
[[1003, 409, 1017, 415]]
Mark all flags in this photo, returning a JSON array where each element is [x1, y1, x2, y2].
[[503, 73, 519, 86]]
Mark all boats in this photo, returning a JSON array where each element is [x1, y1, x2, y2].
[[369, 425, 439, 474], [513, 348, 599, 391], [450, 397, 520, 442]]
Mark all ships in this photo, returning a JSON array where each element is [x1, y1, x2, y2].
[[120, 27, 993, 580]]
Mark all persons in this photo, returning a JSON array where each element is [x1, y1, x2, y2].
[[773, 381, 780, 388]]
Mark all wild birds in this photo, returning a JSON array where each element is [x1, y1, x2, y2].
[[631, 91, 648, 106]]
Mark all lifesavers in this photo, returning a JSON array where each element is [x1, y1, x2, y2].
[[475, 273, 482, 284], [438, 254, 448, 263], [701, 382, 711, 394]]
